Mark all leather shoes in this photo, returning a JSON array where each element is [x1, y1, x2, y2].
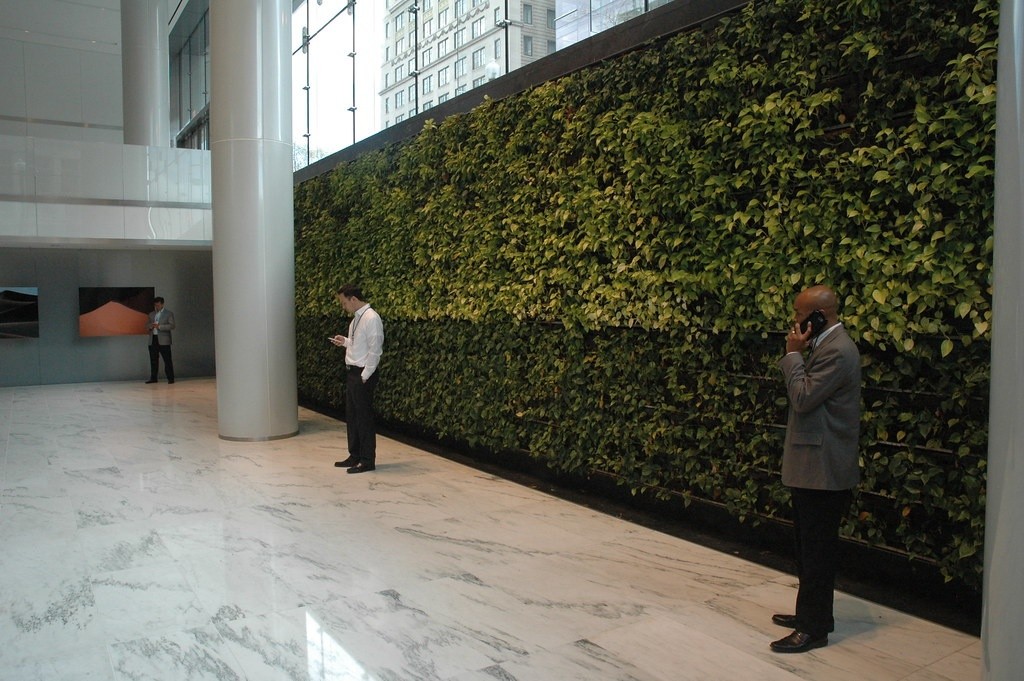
[[770, 631, 830, 654], [335, 456, 375, 474], [772, 614, 836, 633]]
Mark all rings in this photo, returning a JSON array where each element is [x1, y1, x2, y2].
[[791, 330, 795, 333]]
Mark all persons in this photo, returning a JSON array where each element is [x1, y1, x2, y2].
[[145, 297, 175, 385], [331, 283, 385, 473], [770, 285, 863, 655]]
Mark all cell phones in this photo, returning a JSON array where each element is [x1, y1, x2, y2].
[[800, 309, 828, 342], [152, 323, 157, 325], [328, 338, 341, 343]]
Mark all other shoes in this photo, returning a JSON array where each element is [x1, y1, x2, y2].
[[168, 379, 175, 384], [145, 379, 158, 383]]
